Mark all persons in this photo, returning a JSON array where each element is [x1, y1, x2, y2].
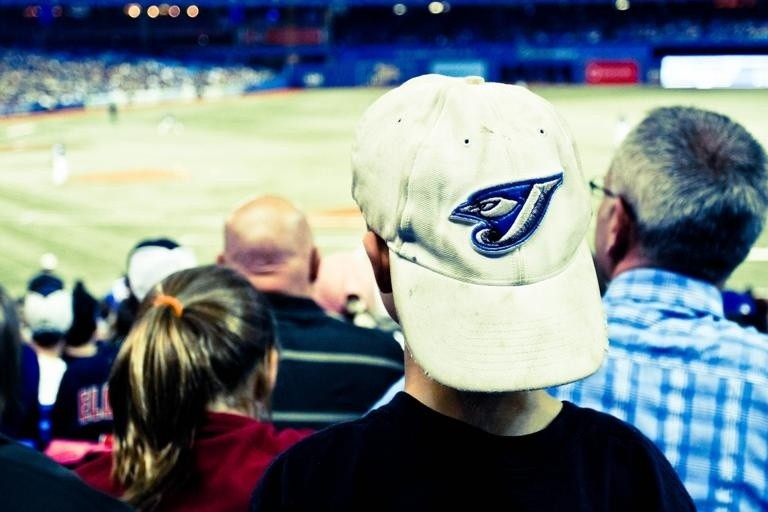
[[246, 74, 699, 512], [0, 286, 136, 512], [21, 235, 196, 469], [216, 195, 406, 417], [106, 263, 313, 512], [370, 106, 768, 512]]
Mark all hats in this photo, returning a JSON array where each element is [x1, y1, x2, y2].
[[351, 73, 609, 395]]
